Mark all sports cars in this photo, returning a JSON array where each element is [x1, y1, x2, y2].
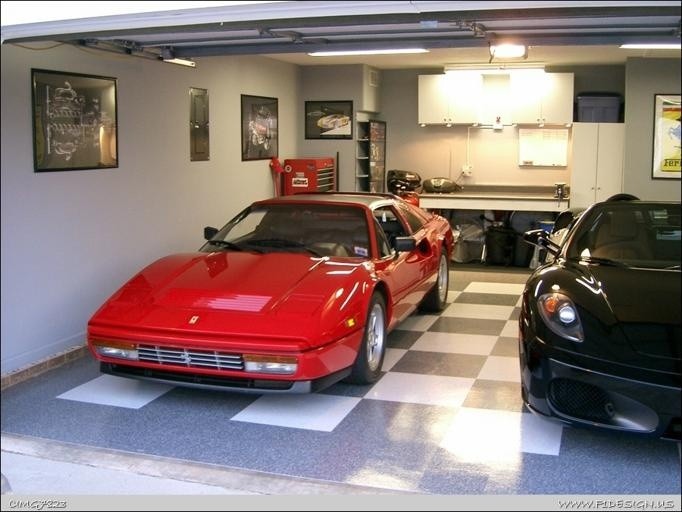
[[514, 201, 682, 442], [86, 192, 453, 394], [316, 114, 351, 130]]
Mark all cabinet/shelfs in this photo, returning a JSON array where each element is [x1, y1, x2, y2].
[[506, 71, 575, 128], [357, 118, 387, 193], [417, 71, 484, 128]]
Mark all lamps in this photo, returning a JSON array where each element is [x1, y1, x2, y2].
[[484, 38, 532, 62]]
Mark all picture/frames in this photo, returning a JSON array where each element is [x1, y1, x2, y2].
[[239, 93, 281, 162], [304, 98, 355, 140], [30, 66, 121, 173], [649, 93, 681, 183]]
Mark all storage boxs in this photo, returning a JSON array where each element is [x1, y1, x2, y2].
[[576, 94, 621, 121]]
